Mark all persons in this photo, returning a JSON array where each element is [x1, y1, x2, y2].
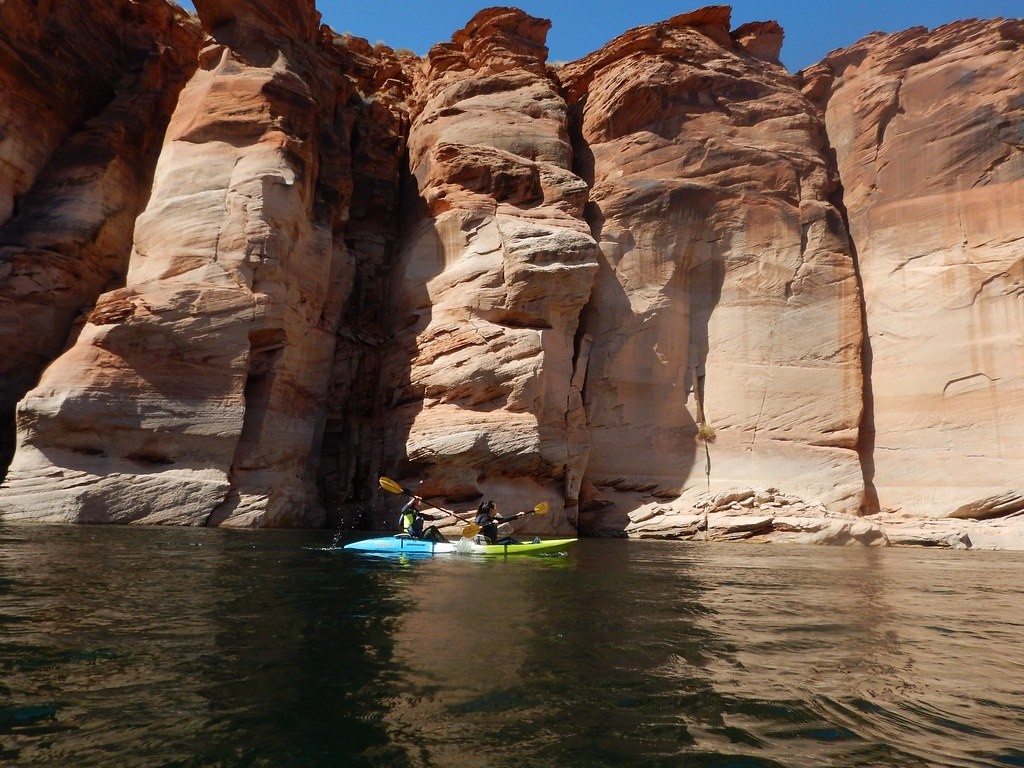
[[401, 495, 454, 543], [474, 500, 541, 544]]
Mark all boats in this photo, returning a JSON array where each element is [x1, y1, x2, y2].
[[343, 528, 579, 556]]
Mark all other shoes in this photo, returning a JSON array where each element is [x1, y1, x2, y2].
[[532, 537, 540, 543]]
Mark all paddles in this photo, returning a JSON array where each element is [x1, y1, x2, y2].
[[379, 475, 471, 526], [461, 501, 549, 540]]
